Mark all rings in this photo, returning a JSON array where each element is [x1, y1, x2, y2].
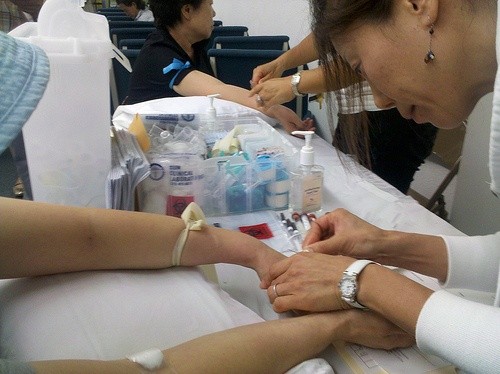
[[273, 283, 278, 298], [250, 80, 253, 85], [257, 95, 261, 101]]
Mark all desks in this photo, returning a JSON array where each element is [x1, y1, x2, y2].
[[186, 125, 500, 374]]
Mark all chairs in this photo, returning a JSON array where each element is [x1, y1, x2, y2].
[[406, 117, 469, 219], [97, 5, 310, 120]]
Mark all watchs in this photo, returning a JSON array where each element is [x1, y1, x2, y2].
[[290, 71, 306, 97], [337, 259, 376, 310]]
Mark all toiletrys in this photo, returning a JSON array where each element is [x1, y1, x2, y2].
[[206, 92, 221, 121], [290, 130, 324, 216]]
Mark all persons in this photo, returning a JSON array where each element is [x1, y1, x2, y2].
[[248, 31, 437, 195], [116, 0, 154, 22], [128, 0, 315, 139], [259, 0, 500, 374], [0, 33, 415, 374]]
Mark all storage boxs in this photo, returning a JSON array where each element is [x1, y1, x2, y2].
[[136, 118, 301, 218]]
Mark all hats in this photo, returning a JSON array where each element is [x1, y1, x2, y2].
[[0, 29, 50, 154]]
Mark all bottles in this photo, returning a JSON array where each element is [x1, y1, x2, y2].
[[6, 0, 116, 209]]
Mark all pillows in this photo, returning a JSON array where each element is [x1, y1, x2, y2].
[[0, 264, 336, 374]]
